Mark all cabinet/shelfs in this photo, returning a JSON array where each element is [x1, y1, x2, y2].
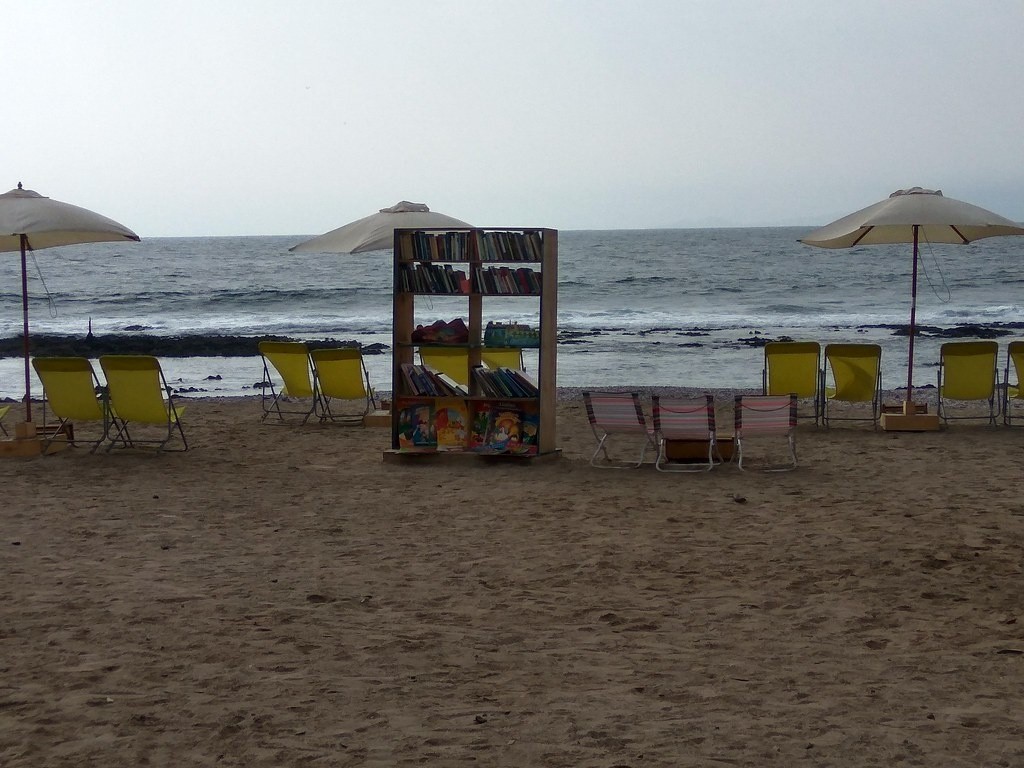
[[392, 227, 558, 455]]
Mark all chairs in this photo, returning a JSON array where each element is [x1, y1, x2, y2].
[[1002, 341, 1024, 428], [822, 343, 883, 434], [763, 342, 822, 431], [99, 354, 188, 454], [258, 341, 322, 427], [730, 392, 798, 473], [420, 346, 526, 387], [651, 393, 723, 473], [32, 358, 127, 454], [311, 348, 376, 425], [937, 341, 1000, 430], [582, 389, 655, 469]]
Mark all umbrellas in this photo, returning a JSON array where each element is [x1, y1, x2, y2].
[[0, 181, 142, 420], [288, 201, 478, 254], [796, 187, 1024, 399]]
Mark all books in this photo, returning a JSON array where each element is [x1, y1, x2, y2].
[[396, 231, 540, 399]]
